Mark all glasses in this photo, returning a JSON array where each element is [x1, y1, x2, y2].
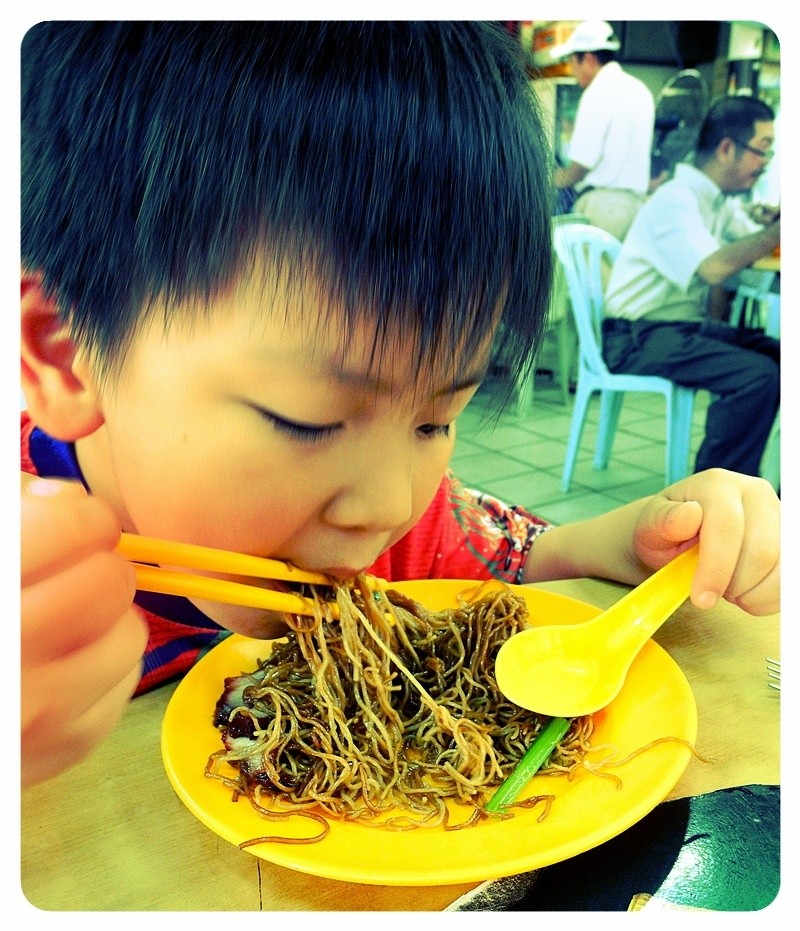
[[736, 140, 775, 163]]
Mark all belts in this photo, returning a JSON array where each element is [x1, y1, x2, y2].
[[578, 186, 594, 197]]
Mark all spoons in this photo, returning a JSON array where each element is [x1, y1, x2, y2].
[[493, 541, 703, 718]]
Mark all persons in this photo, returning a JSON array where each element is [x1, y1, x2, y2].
[[600, 97, 780, 475], [19, 20, 780, 794], [549, 20, 654, 396]]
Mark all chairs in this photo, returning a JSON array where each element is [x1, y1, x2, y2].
[[550, 221, 713, 491]]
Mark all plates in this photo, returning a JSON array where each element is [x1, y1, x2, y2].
[[162, 578, 697, 886]]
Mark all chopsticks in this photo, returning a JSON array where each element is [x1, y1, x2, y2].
[[110, 533, 397, 622]]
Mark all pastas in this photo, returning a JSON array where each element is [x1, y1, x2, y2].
[[203, 562, 716, 851]]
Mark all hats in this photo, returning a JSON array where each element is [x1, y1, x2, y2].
[[549, 21, 620, 59]]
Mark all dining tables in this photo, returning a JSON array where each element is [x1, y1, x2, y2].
[[22, 574, 781, 912]]
